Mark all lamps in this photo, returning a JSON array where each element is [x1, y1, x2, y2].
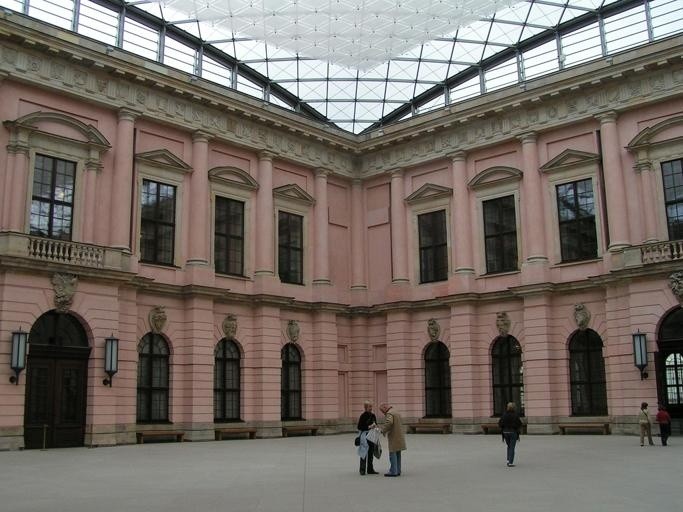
[[10, 325, 28, 386], [103, 332, 120, 387], [633, 328, 648, 380]]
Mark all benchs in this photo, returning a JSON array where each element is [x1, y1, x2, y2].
[[215, 428, 257, 440], [558, 423, 609, 435], [282, 426, 320, 437], [481, 424, 526, 435], [408, 423, 451, 434], [136, 430, 185, 444]]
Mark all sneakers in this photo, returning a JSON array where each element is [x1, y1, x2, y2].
[[360, 471, 364, 475], [367, 472, 378, 474]]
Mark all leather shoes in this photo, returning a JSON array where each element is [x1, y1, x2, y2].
[[383, 474, 396, 477]]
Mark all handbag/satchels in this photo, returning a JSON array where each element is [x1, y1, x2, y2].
[[354, 437, 359, 446]]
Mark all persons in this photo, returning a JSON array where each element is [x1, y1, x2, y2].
[[638, 402, 655, 446], [358, 400, 379, 475], [656, 405, 672, 446], [378, 402, 406, 477], [499, 401, 522, 467]]
